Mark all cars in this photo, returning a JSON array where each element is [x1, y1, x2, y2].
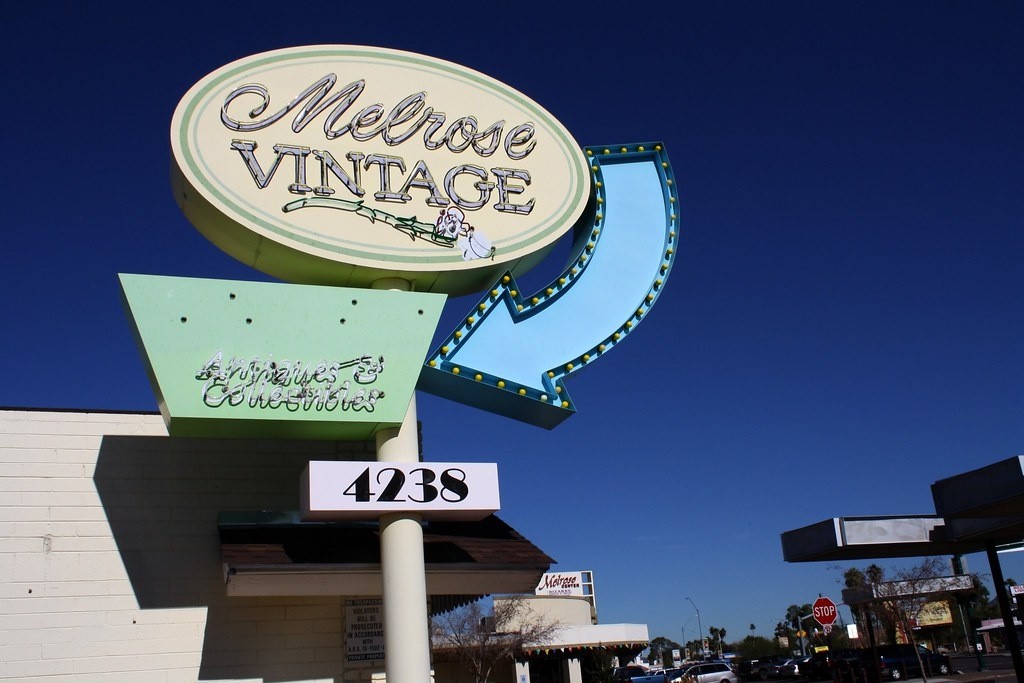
[[807, 648, 859, 680], [671, 663, 738, 683], [854, 643, 949, 682], [649, 657, 813, 680]]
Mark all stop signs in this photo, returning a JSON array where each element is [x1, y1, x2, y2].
[[814, 597, 838, 626]]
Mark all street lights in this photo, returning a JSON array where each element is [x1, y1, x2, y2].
[[685, 597, 702, 639], [682, 614, 698, 648]]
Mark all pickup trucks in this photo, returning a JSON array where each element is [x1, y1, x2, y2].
[[611, 665, 667, 683]]
